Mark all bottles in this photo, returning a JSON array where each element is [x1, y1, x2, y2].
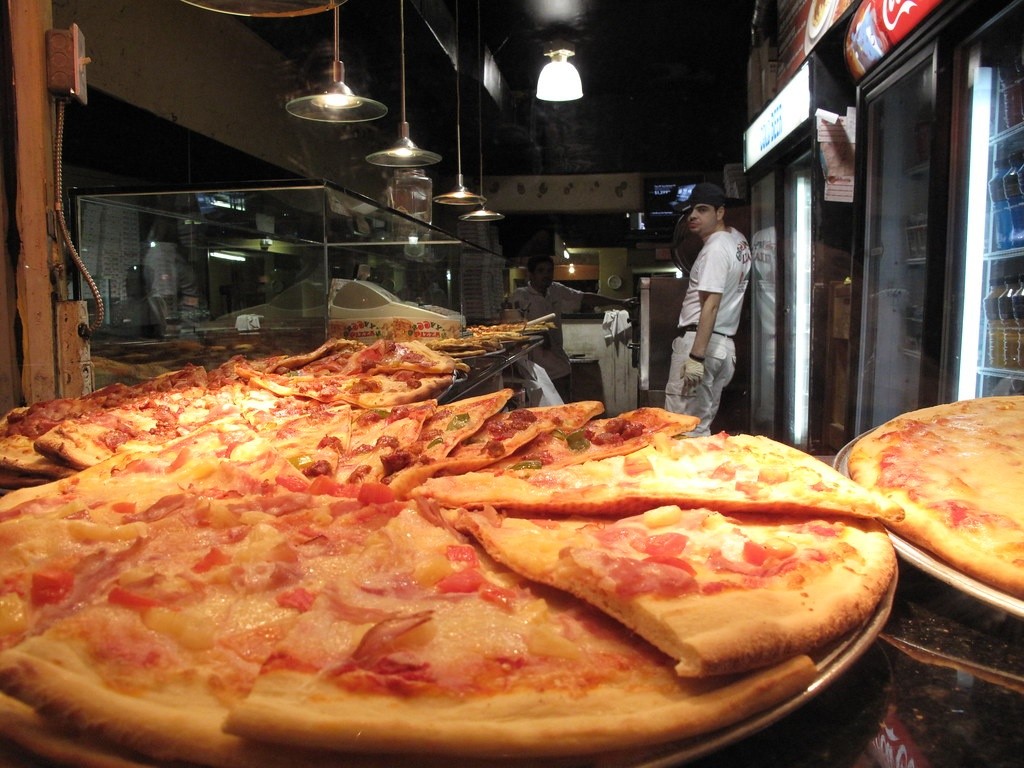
[[983, 276, 1024, 368], [989, 151, 1024, 251], [388, 168, 432, 237], [996, 59, 1024, 128]]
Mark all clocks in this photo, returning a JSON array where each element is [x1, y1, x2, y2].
[[607, 275, 623, 290]]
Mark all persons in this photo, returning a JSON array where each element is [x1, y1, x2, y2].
[[226, 261, 266, 312], [366, 269, 448, 306], [664, 183, 751, 437], [112, 264, 167, 340], [752, 225, 776, 437], [508, 254, 638, 404]]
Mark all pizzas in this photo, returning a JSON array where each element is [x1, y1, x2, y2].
[[0, 314, 906, 768], [847, 394, 1024, 602]]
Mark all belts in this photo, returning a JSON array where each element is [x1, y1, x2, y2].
[[680, 326, 733, 338]]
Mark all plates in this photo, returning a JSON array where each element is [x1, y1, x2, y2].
[[834, 427, 1024, 620], [1, 557, 898, 768]]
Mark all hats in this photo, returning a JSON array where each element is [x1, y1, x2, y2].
[[668, 183, 725, 211]]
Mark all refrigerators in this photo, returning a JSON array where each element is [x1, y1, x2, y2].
[[744, 52, 857, 454], [846, 0, 1024, 443]]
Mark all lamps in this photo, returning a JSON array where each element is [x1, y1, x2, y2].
[[364, 0, 443, 168], [283, 6, 388, 125], [432, 66, 488, 206], [536, 40, 586, 102], [457, 0, 506, 221]]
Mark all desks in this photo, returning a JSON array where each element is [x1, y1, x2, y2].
[[567, 358, 599, 401]]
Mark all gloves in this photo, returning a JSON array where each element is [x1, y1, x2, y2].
[[689, 354, 705, 362]]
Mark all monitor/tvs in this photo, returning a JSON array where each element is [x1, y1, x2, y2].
[[643, 174, 706, 220]]
